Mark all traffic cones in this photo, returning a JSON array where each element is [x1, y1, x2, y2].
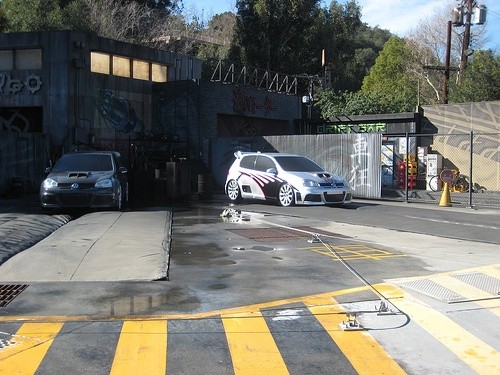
[[439, 182, 453, 208]]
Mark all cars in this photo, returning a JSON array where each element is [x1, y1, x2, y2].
[[37, 151, 128, 214], [224, 150, 353, 209]]
